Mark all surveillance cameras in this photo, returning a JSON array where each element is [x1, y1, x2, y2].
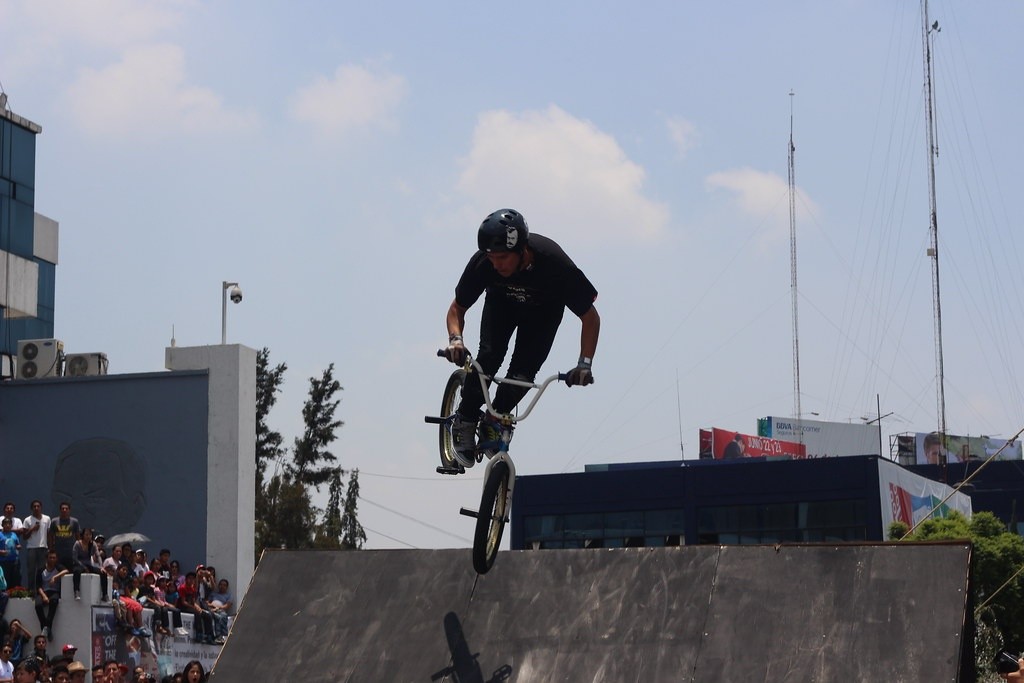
[[230, 286, 242, 303]]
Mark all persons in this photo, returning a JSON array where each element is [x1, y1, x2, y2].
[[35, 551, 69, 642], [23, 500, 51, 597], [924, 434, 940, 463], [162, 659, 210, 683], [1001, 658, 1024, 683], [47, 501, 81, 574], [722, 433, 751, 458], [444, 208, 601, 468], [94, 534, 234, 645], [91, 658, 157, 683], [0, 614, 90, 683], [0, 503, 23, 619], [72, 528, 111, 603]]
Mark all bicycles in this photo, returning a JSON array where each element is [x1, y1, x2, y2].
[[424, 347, 595, 575]]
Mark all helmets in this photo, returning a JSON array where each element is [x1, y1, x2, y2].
[[478, 209, 529, 256]]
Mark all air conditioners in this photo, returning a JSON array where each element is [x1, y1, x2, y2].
[[64, 353, 108, 376], [16, 339, 63, 378]]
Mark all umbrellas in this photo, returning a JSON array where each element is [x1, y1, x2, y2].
[[103, 532, 151, 548]]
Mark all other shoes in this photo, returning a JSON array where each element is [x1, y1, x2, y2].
[[157, 627, 167, 634], [166, 630, 175, 637], [137, 628, 150, 637], [174, 628, 188, 638], [129, 628, 139, 636]]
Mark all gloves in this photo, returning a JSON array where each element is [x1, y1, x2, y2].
[[565, 362, 592, 387], [445, 336, 471, 368]]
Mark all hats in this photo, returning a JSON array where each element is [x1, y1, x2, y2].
[[94, 535, 105, 541], [158, 576, 167, 581], [196, 564, 204, 572], [144, 571, 154, 580], [63, 645, 77, 653], [137, 549, 144, 554]]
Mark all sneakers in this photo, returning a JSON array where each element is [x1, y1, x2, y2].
[[479, 424, 500, 459], [450, 418, 477, 468]]
